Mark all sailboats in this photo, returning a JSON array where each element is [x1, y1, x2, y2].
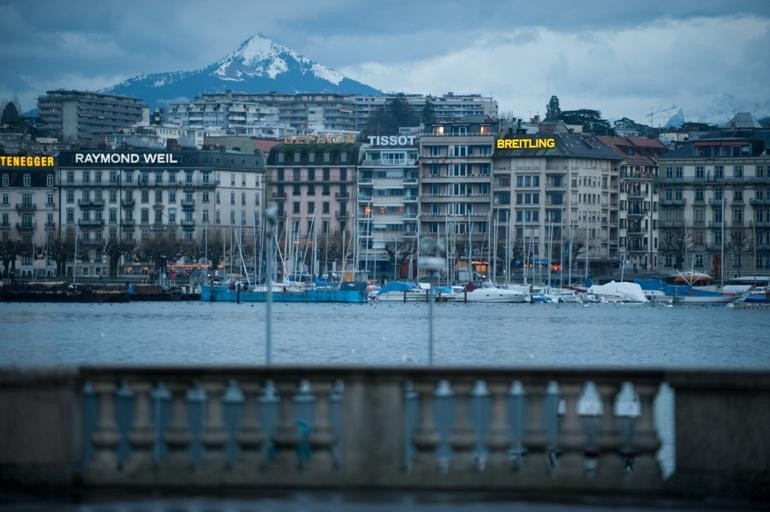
[[0, 198, 770, 307]]
